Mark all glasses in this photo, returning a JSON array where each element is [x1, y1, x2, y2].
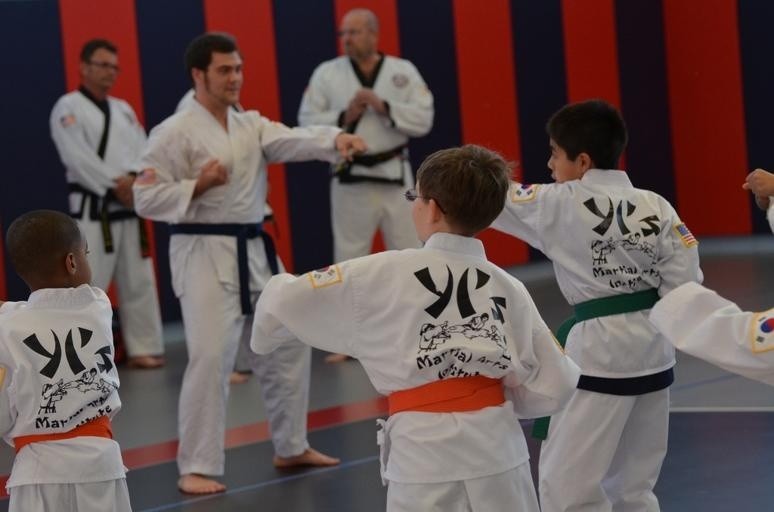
[[91, 60, 118, 71], [404, 189, 446, 217]]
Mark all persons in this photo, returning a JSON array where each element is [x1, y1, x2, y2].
[[130, 34, 368, 496], [742, 168, 774, 233], [298, 8, 436, 362], [0, 208, 133, 512], [250, 144, 582, 512], [48, 39, 169, 368], [650, 281, 774, 387], [175, 89, 253, 388], [487, 97, 705, 512]]
